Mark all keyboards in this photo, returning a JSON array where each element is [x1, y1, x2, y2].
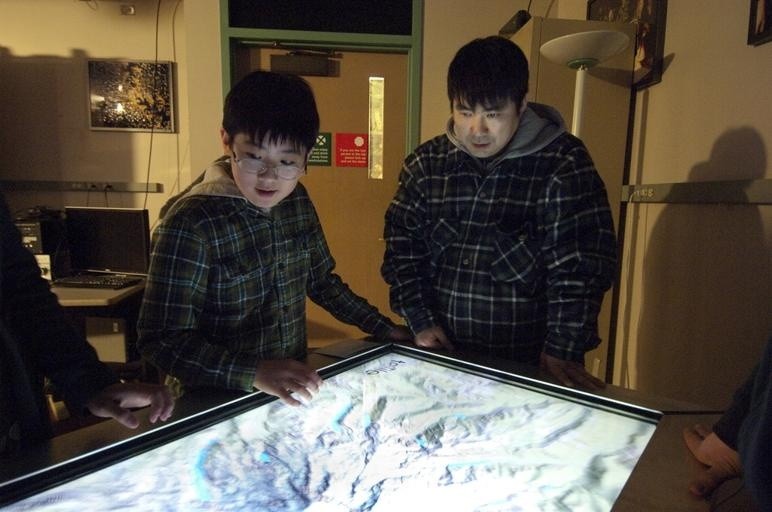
[[54, 275, 142, 289]]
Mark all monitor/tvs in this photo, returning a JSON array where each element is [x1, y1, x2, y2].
[[64, 205, 150, 276]]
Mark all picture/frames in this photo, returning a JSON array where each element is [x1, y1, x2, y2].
[[85, 58, 175, 134], [747, 1, 771, 47], [586, 1, 668, 92]]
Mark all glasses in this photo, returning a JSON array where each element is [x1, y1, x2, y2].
[[228, 133, 308, 181]]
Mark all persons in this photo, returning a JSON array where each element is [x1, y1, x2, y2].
[[379, 36, 621, 390], [133, 66, 411, 410], [682, 334, 769, 510], [0, 195, 176, 453]]
